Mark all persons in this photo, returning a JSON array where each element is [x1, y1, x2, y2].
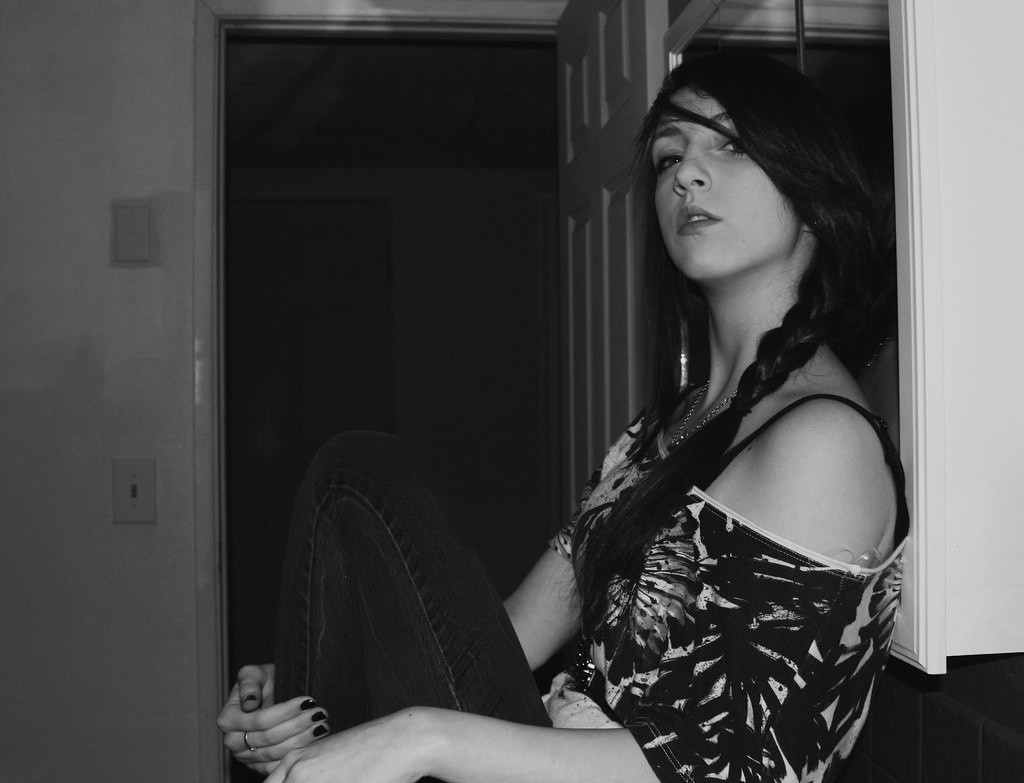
[[828, 85, 900, 456], [217, 49, 909, 783]]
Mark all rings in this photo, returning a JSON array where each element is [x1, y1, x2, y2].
[[244, 732, 257, 752]]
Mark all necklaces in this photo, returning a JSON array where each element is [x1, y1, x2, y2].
[[863, 335, 898, 368], [571, 381, 737, 693]]
